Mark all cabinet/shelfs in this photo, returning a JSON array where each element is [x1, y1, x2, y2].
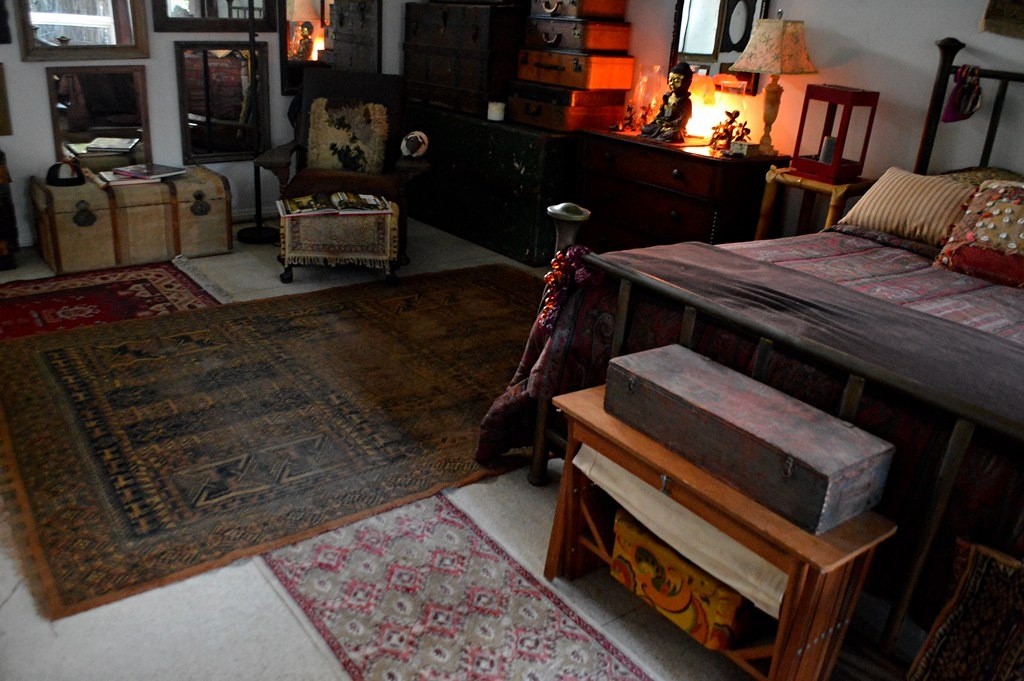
[[544, 388, 903, 681]]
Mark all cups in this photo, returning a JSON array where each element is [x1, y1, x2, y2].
[[487, 108, 505, 121]]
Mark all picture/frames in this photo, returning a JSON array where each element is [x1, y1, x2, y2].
[[46, 64, 152, 173], [174, 40, 272, 166], [150, 0, 286, 33]]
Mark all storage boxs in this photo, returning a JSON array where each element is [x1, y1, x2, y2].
[[602, 343, 896, 534], [404, 0, 522, 115], [332, 0, 382, 75], [28, 162, 234, 275]]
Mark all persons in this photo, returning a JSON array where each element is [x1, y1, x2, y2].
[[642, 60, 693, 141], [294, 21, 314, 60]]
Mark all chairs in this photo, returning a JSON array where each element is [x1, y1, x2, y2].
[[254, 66, 412, 281]]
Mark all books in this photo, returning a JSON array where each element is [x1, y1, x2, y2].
[[86, 136, 140, 151], [276, 192, 395, 217], [99, 170, 161, 185], [112, 162, 187, 179]]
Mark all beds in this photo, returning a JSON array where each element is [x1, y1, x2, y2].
[[474, 31, 1024, 543]]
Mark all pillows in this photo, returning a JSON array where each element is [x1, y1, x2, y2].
[[837, 166, 1024, 290]]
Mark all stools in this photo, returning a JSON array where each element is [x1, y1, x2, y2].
[[752, 163, 880, 239]]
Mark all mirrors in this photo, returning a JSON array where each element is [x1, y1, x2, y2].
[[12, 0, 151, 62], [678, 0, 728, 62], [277, 0, 383, 97]]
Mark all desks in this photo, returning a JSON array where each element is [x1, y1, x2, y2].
[[382, 96, 588, 267], [578, 124, 791, 244]]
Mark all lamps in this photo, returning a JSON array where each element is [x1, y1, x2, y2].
[[728, 8, 818, 158]]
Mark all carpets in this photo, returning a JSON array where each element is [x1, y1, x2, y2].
[[0, 259, 654, 681]]
